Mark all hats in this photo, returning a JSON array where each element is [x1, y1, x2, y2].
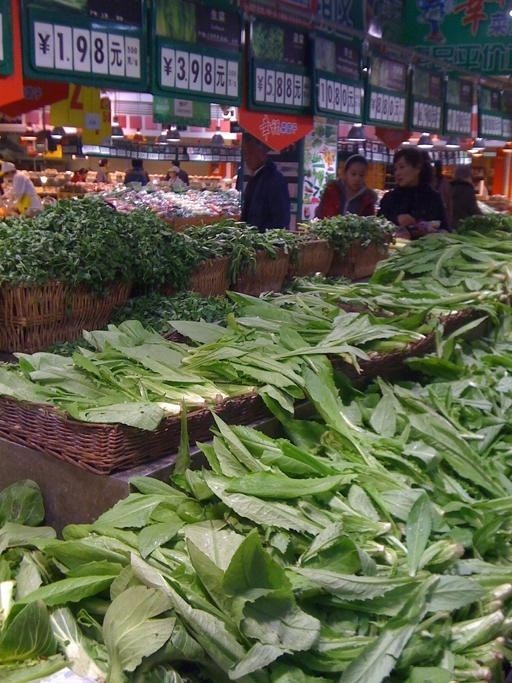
[[0, 161, 15, 176], [168, 166, 181, 173]]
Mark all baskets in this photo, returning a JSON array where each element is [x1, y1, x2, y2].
[[0, 350, 227, 475], [136, 252, 233, 305], [228, 240, 290, 298], [286, 237, 338, 285], [0, 267, 136, 363], [161, 325, 311, 431], [330, 228, 391, 283], [320, 328, 437, 392], [336, 292, 493, 348]]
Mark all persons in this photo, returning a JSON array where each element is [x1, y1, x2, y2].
[[164, 159, 190, 187], [430, 159, 455, 227], [0, 160, 44, 213], [449, 162, 486, 221], [375, 146, 453, 233], [72, 167, 89, 182], [238, 139, 292, 228], [94, 157, 111, 183], [168, 166, 183, 183], [473, 167, 488, 201], [313, 152, 378, 218], [123, 158, 149, 186]]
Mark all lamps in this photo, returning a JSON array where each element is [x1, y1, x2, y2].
[[20, 95, 243, 153], [343, 120, 512, 160]]
